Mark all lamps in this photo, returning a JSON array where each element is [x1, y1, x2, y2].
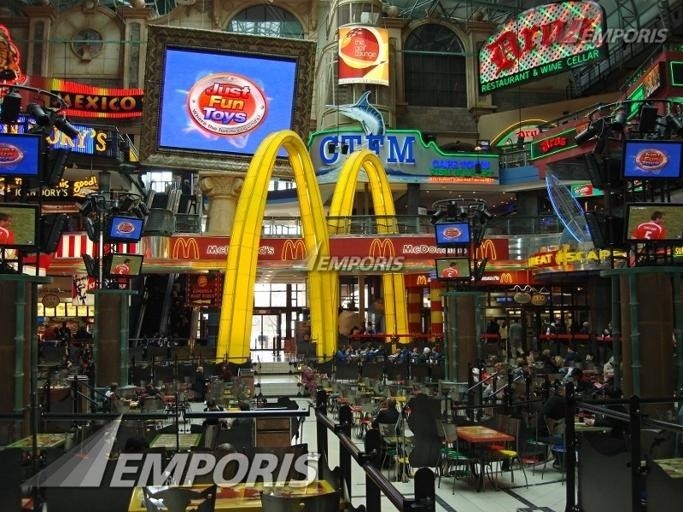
[[474, 259, 488, 281], [0, 69, 80, 140], [586, 211, 609, 250], [516, 0, 525, 149], [119, 140, 126, 151], [105, 138, 112, 150], [475, 1, 482, 174], [430, 200, 494, 224], [328, 142, 336, 153], [574, 96, 683, 157], [77, 190, 150, 219], [341, 144, 348, 154], [39, 213, 67, 253]]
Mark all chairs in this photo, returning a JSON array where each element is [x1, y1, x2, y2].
[[290, 360, 565, 495], [105, 382, 194, 432], [259, 488, 342, 512], [142, 484, 217, 512]]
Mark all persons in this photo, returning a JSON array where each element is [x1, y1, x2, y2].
[[292, 313, 626, 485], [41, 283, 254, 430], [0, 212, 15, 244], [440, 262, 459, 278], [110, 254, 133, 276], [630, 207, 668, 239]]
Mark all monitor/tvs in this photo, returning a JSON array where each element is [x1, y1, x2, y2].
[[0, 132, 42, 175], [620, 137, 683, 182], [0, 202, 43, 249], [435, 257, 470, 280], [105, 252, 144, 276], [624, 202, 683, 244], [436, 222, 470, 248], [107, 216, 145, 245]]
